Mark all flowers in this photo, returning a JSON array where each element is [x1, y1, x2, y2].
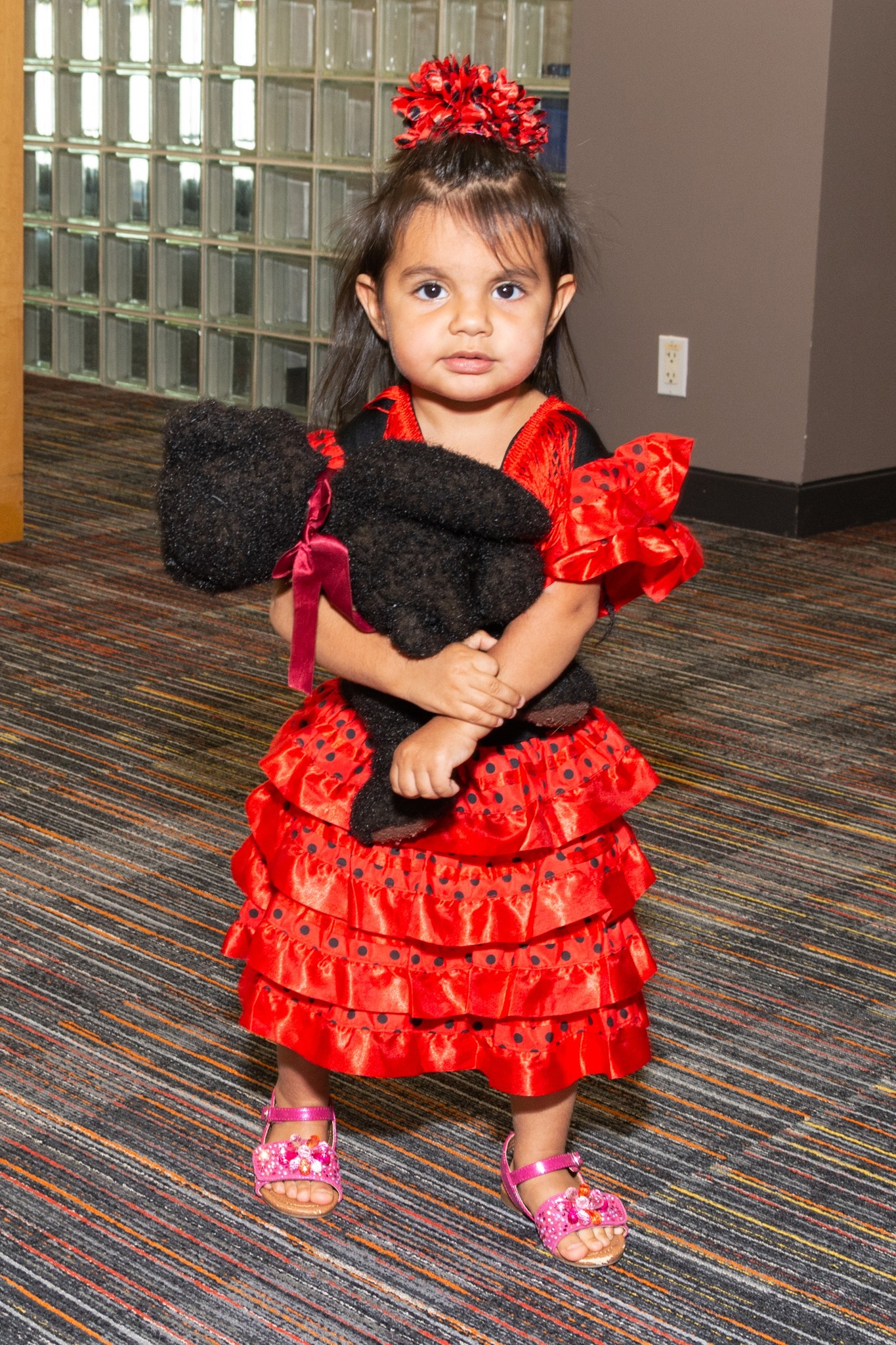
[[388, 52, 555, 155]]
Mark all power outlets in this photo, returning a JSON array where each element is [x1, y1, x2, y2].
[[650, 325, 693, 400]]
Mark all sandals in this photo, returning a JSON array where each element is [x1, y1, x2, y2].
[[249, 1082, 343, 1218], [497, 1130, 630, 1270]]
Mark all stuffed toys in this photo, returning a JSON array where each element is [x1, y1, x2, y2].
[[158, 398, 597, 846]]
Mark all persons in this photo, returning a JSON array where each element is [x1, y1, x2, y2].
[[224, 53, 704, 1269]]
